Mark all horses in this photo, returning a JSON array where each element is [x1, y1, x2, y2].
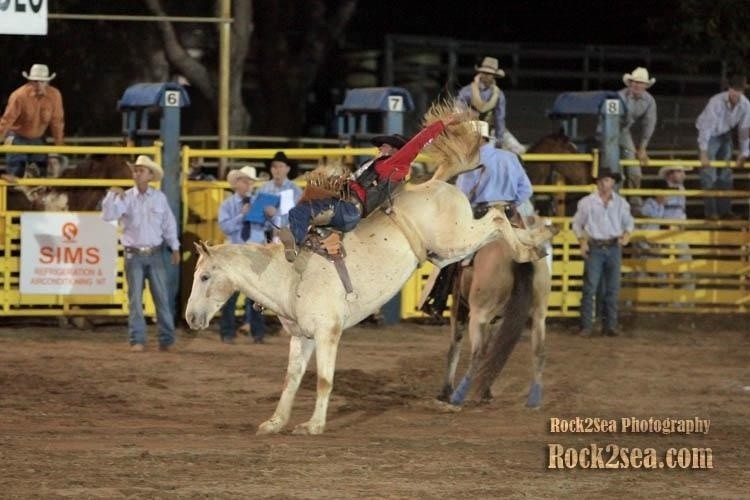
[[185, 82, 561, 435], [524, 131, 587, 185], [440, 233, 553, 408], [7, 142, 132, 210]]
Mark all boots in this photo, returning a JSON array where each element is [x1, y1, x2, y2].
[[421, 264, 458, 322]]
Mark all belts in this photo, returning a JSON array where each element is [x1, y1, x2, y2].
[[125, 247, 163, 254], [589, 240, 616, 245]]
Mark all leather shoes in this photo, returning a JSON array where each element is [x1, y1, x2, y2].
[[254, 338, 263, 344], [131, 344, 145, 352], [160, 345, 179, 352], [279, 226, 297, 261], [221, 336, 232, 343]]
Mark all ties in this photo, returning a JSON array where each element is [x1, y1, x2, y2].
[[240, 197, 251, 242]]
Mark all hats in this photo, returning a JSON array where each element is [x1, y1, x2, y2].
[[469, 120, 495, 140], [657, 166, 694, 179], [474, 56, 506, 78], [726, 71, 750, 90], [22, 64, 56, 82], [370, 134, 408, 150], [590, 167, 622, 184], [265, 152, 297, 173], [622, 67, 656, 89], [227, 166, 266, 186], [126, 155, 163, 182]]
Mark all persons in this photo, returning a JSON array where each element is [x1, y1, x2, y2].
[[0, 63, 70, 184], [253, 151, 305, 244], [279, 115, 459, 261], [628, 180, 681, 306], [103, 154, 181, 353], [595, 67, 657, 204], [657, 165, 697, 308], [452, 57, 507, 149], [695, 76, 750, 220], [422, 118, 533, 321], [573, 168, 635, 336], [218, 165, 267, 344]]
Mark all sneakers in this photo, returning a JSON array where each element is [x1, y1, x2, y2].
[[721, 213, 741, 220], [704, 215, 720, 220], [579, 330, 592, 338], [602, 328, 619, 337]]
